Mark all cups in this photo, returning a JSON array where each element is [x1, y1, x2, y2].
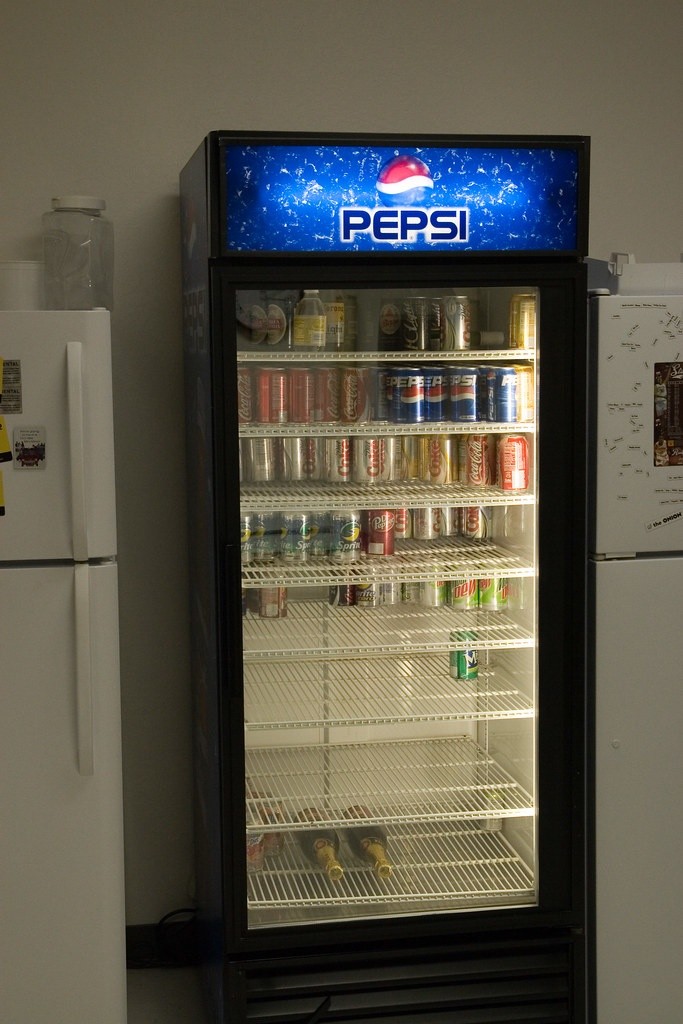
[[0, 259, 45, 310]]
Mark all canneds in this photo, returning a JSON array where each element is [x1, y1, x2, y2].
[[247, 793, 285, 873], [450, 626, 478, 680], [238, 363, 534, 618], [377, 293, 538, 351], [478, 787, 503, 833], [249, 293, 358, 352]]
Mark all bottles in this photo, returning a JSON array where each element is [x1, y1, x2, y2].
[[40, 196, 115, 309], [293, 808, 343, 881], [342, 806, 391, 878], [236, 290, 357, 350]]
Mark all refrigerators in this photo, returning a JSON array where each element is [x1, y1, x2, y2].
[[0, 307, 127, 1024], [175, 128, 593, 1024], [589, 258, 683, 1023]]
